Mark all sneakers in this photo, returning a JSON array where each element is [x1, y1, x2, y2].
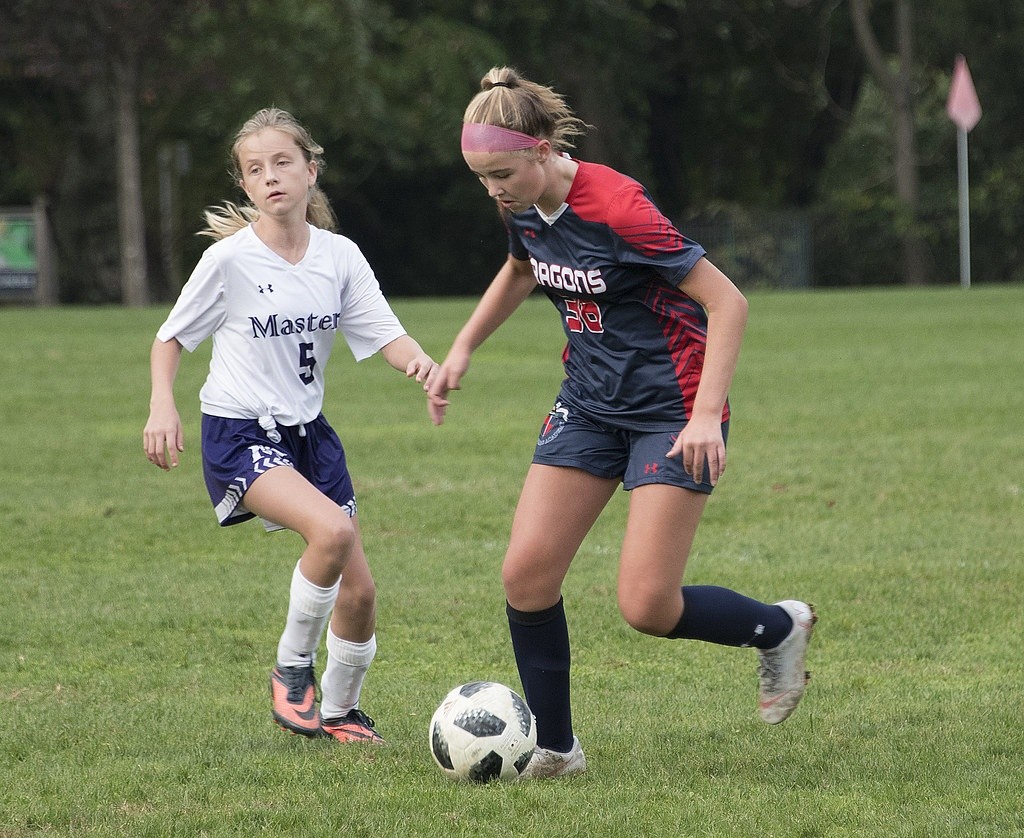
[[271, 663, 321, 735], [319, 709, 386, 746], [517, 735, 586, 778], [754, 600, 818, 725]]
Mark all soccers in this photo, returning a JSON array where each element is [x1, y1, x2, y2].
[[428, 680, 538, 786]]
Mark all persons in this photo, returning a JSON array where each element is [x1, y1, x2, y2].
[[143, 110, 440, 744], [426, 69, 816, 781]]
